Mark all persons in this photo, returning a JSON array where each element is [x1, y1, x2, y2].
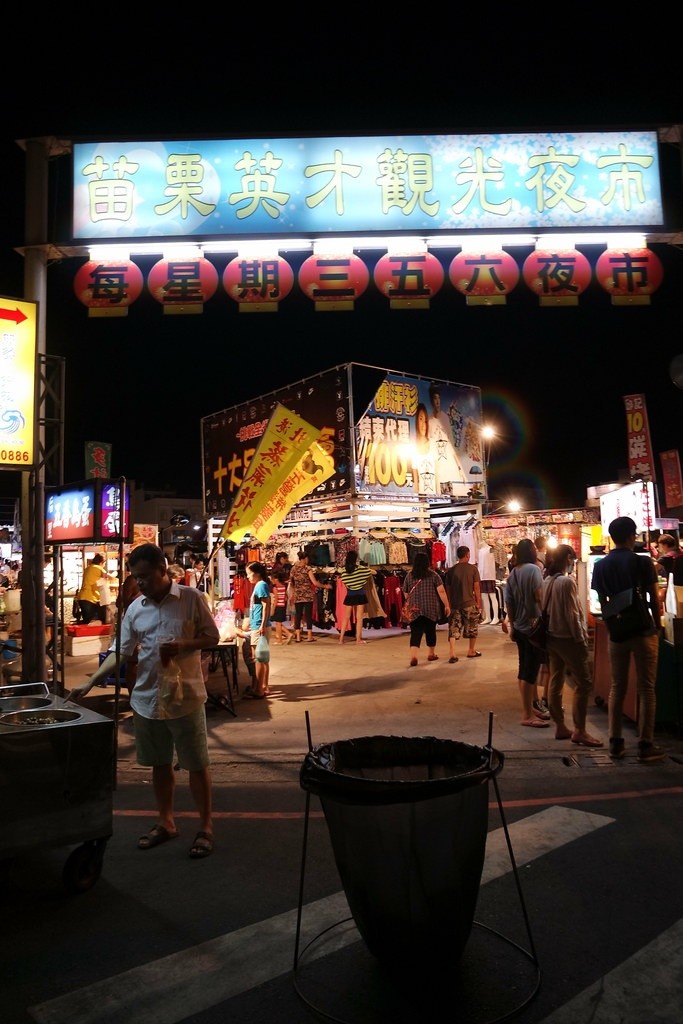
[[75, 553, 212, 624], [338, 550, 378, 645], [592, 517, 667, 762], [445, 546, 482, 663], [0, 548, 63, 641], [411, 382, 468, 503], [540, 544, 603, 745], [290, 551, 333, 642], [244, 560, 272, 698], [654, 534, 683, 586], [503, 537, 551, 727], [235, 618, 257, 691], [437, 513, 482, 569], [477, 539, 508, 625], [64, 543, 220, 857], [233, 551, 294, 645], [402, 552, 451, 666]]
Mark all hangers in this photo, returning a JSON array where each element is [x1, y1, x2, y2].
[[326, 566, 411, 582], [441, 516, 481, 536], [244, 532, 442, 544]]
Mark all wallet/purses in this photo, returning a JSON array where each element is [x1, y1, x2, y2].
[[252, 594, 272, 604]]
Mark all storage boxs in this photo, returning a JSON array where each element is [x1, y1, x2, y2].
[[3, 590, 22, 632], [64, 623, 112, 657], [100, 651, 124, 689]]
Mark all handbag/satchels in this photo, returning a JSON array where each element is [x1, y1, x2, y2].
[[527, 610, 550, 653]]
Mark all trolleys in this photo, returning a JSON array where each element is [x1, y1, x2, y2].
[[0, 476, 125, 896], [586, 481, 683, 737]]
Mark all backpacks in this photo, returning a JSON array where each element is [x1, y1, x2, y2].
[[599, 556, 654, 640]]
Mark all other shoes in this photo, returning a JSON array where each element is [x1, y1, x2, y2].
[[356, 640, 368, 644], [448, 656, 459, 664], [410, 658, 417, 667], [555, 731, 575, 740], [523, 697, 565, 728], [571, 734, 602, 748], [467, 651, 481, 658], [242, 687, 269, 699], [427, 654, 439, 661], [272, 627, 316, 645], [606, 737, 624, 759], [338, 640, 345, 645], [635, 743, 667, 761]]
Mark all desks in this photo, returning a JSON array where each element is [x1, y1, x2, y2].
[[199, 639, 240, 718]]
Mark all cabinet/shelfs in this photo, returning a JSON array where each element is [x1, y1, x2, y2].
[[584, 599, 683, 741]]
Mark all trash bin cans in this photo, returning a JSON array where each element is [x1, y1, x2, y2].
[[303, 736, 503, 971]]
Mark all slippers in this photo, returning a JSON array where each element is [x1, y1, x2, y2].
[[188, 832, 216, 857], [138, 824, 180, 848]]
